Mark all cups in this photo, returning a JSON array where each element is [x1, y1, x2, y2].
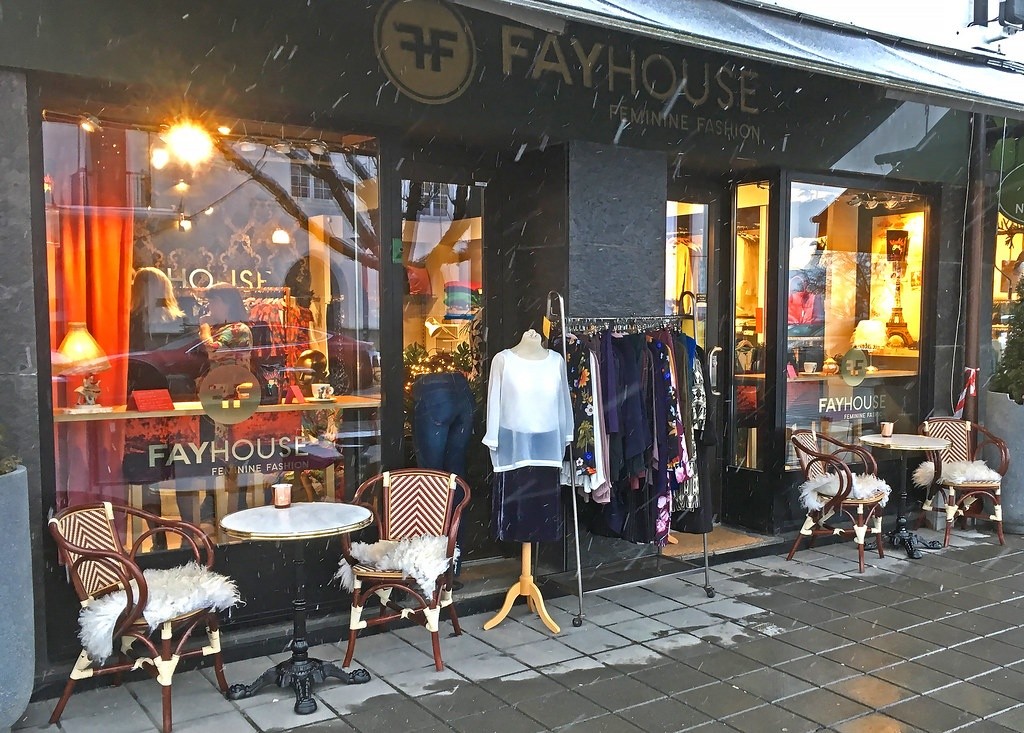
[[271, 483, 293, 509], [804, 362, 817, 373], [311, 383, 334, 399], [881, 422, 894, 437]]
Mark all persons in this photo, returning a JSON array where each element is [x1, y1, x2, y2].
[[414, 371, 475, 571], [485, 329, 574, 541], [124, 265, 255, 550]]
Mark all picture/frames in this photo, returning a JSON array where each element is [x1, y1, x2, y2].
[[854, 199, 931, 358], [992, 228, 1024, 304]]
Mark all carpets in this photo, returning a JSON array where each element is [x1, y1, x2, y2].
[[662, 526, 763, 556]]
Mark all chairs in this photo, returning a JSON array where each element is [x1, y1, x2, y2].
[[49, 502, 229, 733], [342, 468, 472, 671], [787, 429, 892, 573], [918, 417, 1011, 549]]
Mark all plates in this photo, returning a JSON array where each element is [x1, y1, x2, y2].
[[799, 372, 821, 376], [305, 397, 337, 402]]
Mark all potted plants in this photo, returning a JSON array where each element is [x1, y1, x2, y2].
[[982, 273, 1024, 534], [0, 454, 36, 728]]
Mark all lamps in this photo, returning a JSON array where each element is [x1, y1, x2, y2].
[[306, 131, 328, 155], [850, 321, 887, 372], [270, 125, 293, 153], [50, 322, 112, 411], [78, 107, 106, 133], [236, 123, 257, 151], [425, 317, 458, 342]]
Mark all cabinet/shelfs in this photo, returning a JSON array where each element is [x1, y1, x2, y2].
[[308, 214, 482, 502]]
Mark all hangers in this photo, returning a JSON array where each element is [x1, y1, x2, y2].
[[736, 331, 755, 350], [243, 286, 300, 310], [736, 226, 760, 247], [668, 227, 703, 253]]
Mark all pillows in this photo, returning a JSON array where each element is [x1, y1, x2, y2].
[[790, 235, 827, 270], [404, 265, 432, 294]]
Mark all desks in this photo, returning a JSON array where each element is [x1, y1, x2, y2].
[[859, 434, 953, 559], [220, 501, 375, 715], [53, 396, 381, 565], [735, 369, 918, 469]]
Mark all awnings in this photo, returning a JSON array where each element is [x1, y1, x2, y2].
[[506, 1, 1024, 115]]
